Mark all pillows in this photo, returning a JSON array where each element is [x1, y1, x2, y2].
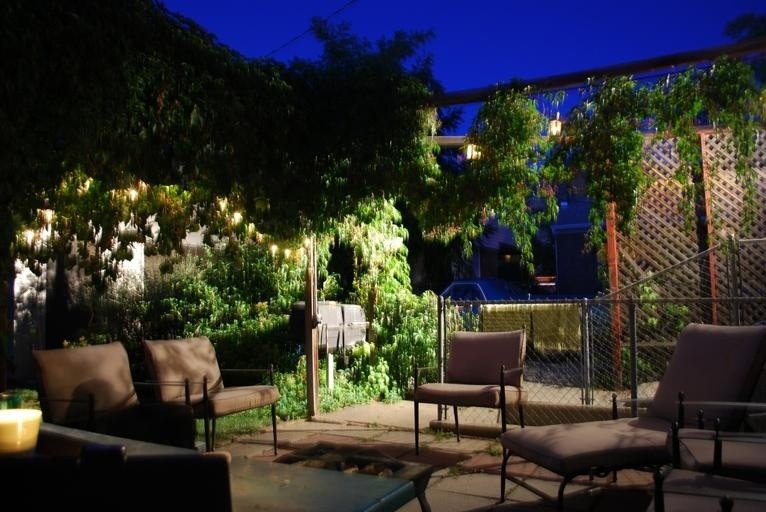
[[647, 324, 765, 431]]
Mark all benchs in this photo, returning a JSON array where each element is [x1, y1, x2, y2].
[[35, 333, 282, 463]]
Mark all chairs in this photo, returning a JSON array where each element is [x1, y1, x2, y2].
[[653, 392, 765, 511], [502, 323, 764, 508], [412, 327, 527, 458]]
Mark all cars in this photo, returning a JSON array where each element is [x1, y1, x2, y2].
[[439, 277, 535, 328]]
